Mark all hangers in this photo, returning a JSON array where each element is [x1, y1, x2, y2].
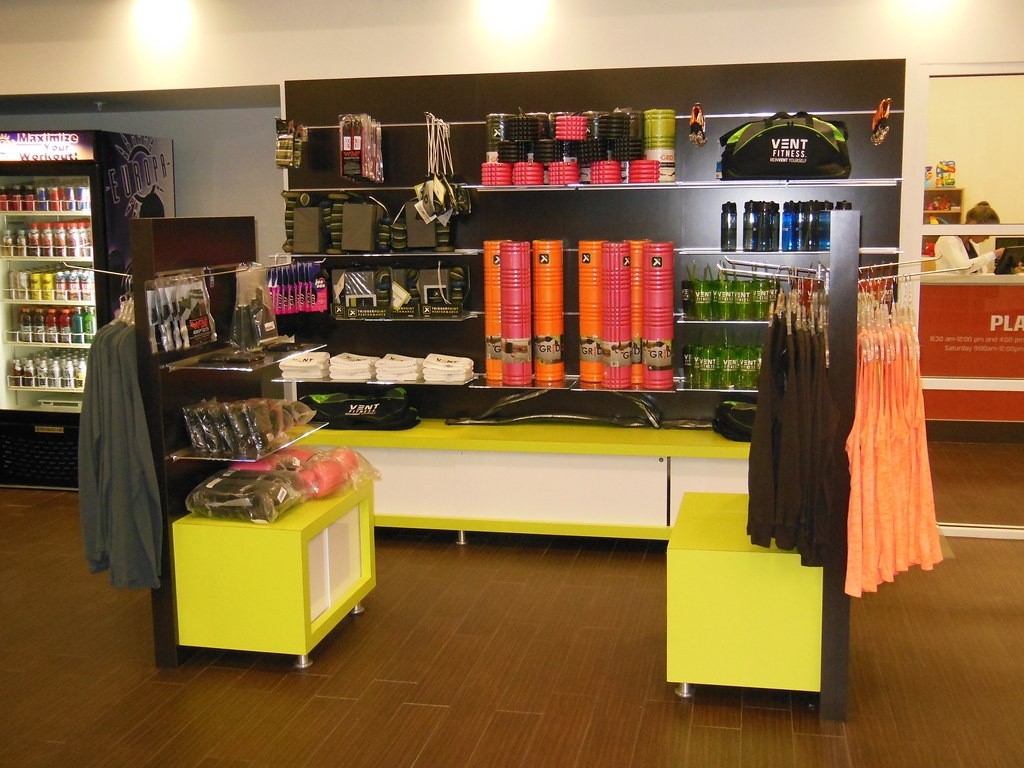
[[767, 273, 921, 368], [111, 273, 135, 326]]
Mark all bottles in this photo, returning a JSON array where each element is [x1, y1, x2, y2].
[[743, 200, 779, 251], [721, 202, 736, 251], [3, 218, 92, 257], [0, 185, 35, 210], [11, 349, 89, 388], [16, 305, 95, 343], [782, 200, 852, 251]]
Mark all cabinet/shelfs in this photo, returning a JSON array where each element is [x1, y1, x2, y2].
[[169, 477, 378, 669], [131, 213, 330, 674], [286, 418, 751, 547], [270, 97, 902, 394], [663, 489, 823, 702], [922, 186, 965, 256]]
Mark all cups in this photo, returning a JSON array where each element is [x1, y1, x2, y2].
[[683, 344, 764, 386], [682, 280, 774, 319]]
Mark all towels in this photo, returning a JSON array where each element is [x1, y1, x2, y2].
[[279, 354, 477, 381]]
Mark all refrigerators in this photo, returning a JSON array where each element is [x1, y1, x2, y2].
[[0, 129, 176, 491]]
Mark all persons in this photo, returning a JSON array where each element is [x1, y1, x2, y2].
[[933, 201, 1005, 274]]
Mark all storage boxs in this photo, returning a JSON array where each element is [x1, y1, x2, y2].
[[341, 203, 384, 251], [330, 268, 390, 319], [391, 267, 465, 319], [404, 201, 438, 248], [291, 207, 330, 253]]
